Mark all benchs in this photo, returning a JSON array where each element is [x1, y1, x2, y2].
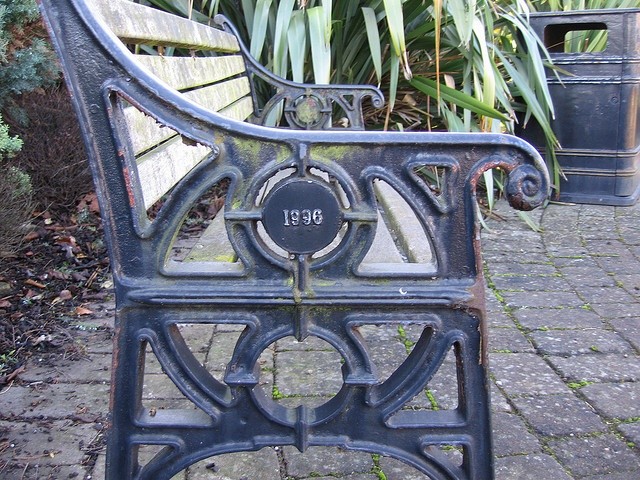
[[37, 0, 553, 480]]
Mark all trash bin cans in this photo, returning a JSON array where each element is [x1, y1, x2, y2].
[[517, 8, 640, 207]]
